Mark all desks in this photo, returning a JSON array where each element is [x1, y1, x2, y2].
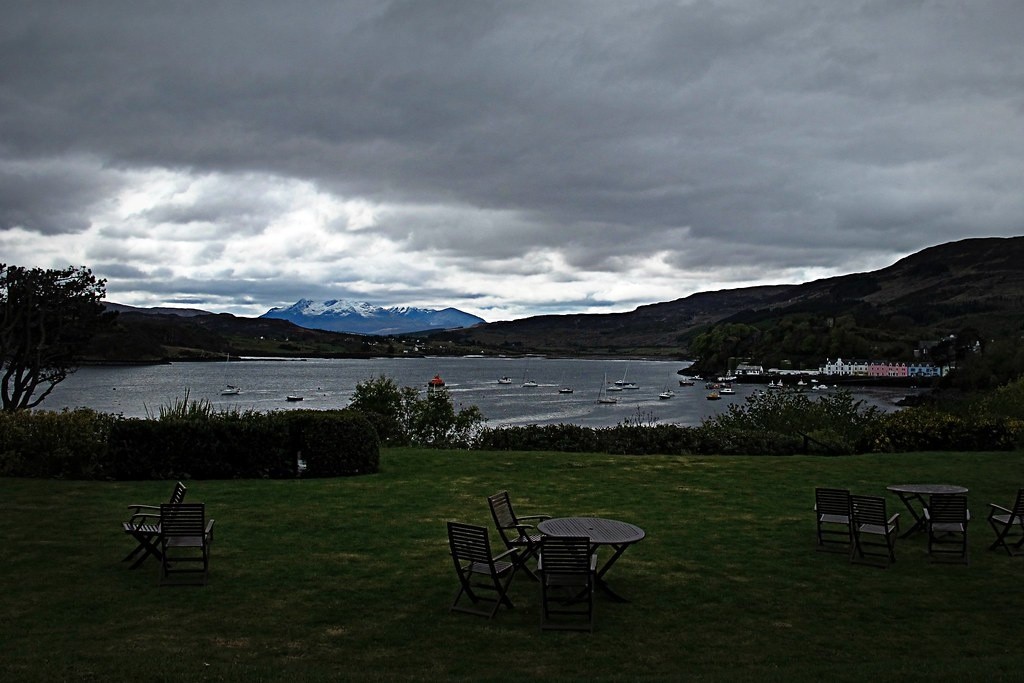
[[885, 484, 969, 545], [536, 517, 646, 604]]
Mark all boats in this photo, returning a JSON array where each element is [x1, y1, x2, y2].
[[428, 375, 445, 386], [622, 383, 639, 389], [719, 388, 736, 394], [523, 380, 538, 388], [614, 361, 631, 385], [287, 395, 304, 402], [665, 390, 677, 396], [658, 393, 670, 399], [220, 353, 240, 396], [679, 380, 695, 386], [498, 376, 512, 384], [704, 391, 721, 400], [559, 387, 575, 393], [706, 384, 731, 389], [598, 372, 618, 403], [606, 386, 623, 391]]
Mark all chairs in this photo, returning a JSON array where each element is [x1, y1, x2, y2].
[[120, 480, 187, 569], [922, 494, 971, 564], [814, 487, 858, 553], [850, 494, 900, 569], [537, 534, 598, 633], [447, 521, 522, 620], [159, 502, 215, 586], [488, 489, 552, 583], [986, 488, 1024, 557]]
